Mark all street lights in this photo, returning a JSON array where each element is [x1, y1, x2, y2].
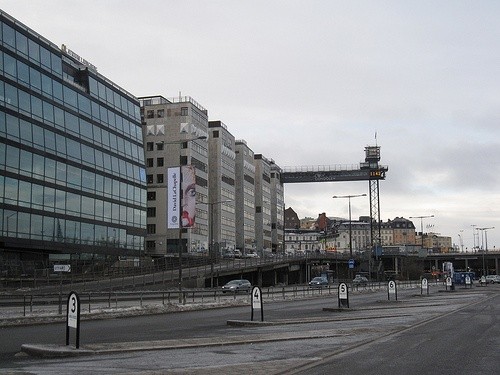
[[152, 136, 207, 305], [332, 193, 366, 281], [471, 224, 495, 255], [409, 215, 435, 249], [196, 200, 233, 287]]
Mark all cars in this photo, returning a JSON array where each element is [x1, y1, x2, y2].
[[222, 279, 251, 293], [352, 276, 369, 285], [479, 275, 500, 284]]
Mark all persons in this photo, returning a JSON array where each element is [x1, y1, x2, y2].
[[180, 166, 195, 228]]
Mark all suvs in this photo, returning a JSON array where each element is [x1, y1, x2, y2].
[[309, 277, 329, 289]]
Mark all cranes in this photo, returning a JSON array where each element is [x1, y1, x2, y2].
[[281, 144, 389, 282]]
